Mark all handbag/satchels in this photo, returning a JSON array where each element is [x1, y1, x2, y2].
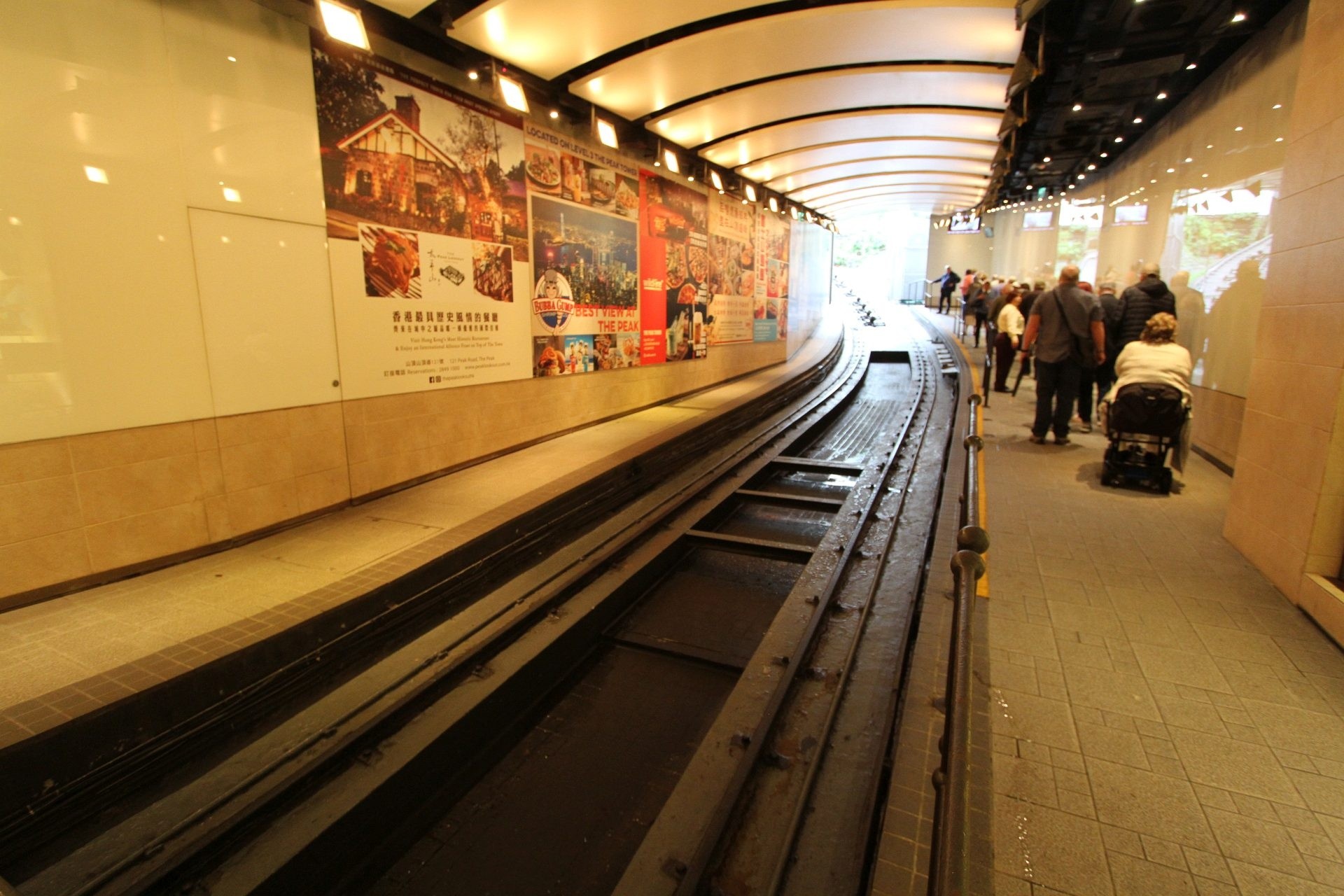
[[1070, 334, 1098, 369]]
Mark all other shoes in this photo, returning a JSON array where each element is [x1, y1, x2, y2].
[[994, 384, 1013, 393], [1030, 434, 1046, 445], [1054, 437, 1070, 445], [1080, 422, 1092, 432]]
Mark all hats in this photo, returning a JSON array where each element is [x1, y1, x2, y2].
[[1145, 263, 1160, 275], [1100, 282, 1115, 292]]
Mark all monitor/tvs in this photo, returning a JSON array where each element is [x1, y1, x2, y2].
[[949, 214, 982, 234]]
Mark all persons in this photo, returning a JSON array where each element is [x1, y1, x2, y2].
[[1072, 281, 1094, 433], [1019, 264, 1106, 446], [961, 268, 1046, 392], [1111, 263, 1177, 388], [1201, 258, 1264, 397], [1167, 271, 1206, 385], [930, 265, 961, 315], [1096, 281, 1118, 426], [1098, 312, 1195, 477]]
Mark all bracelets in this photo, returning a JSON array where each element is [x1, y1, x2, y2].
[[1098, 350, 1105, 353], [1019, 349, 1029, 352]]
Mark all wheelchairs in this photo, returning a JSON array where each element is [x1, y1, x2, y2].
[[1101, 381, 1191, 495]]
